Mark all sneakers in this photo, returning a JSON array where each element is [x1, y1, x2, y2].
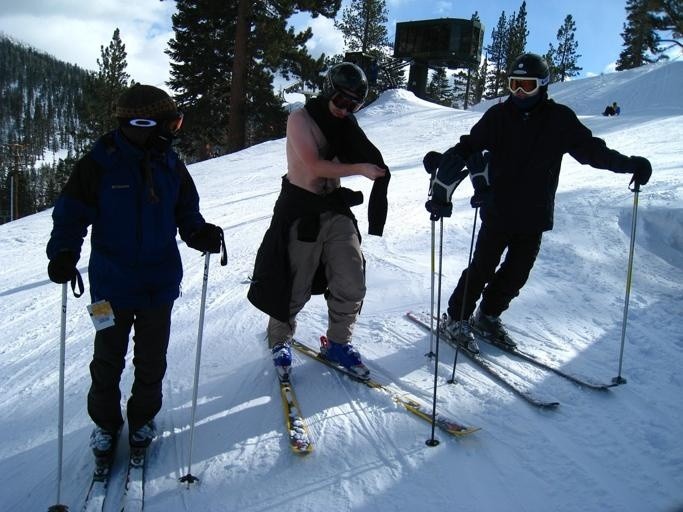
[[272, 342, 292, 381], [89, 421, 156, 458], [320, 336, 370, 376], [445, 307, 517, 354]]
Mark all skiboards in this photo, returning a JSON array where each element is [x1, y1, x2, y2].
[[407, 310, 617, 409], [273, 338, 482, 455], [81, 447, 147, 511]]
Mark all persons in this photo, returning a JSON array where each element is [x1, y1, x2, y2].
[[602, 102, 620, 118], [246, 61, 387, 382], [46, 81, 221, 458], [422, 52, 654, 355]]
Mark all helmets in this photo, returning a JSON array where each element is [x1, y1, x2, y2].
[[509, 54, 549, 95], [116, 83, 183, 143], [322, 62, 368, 113]]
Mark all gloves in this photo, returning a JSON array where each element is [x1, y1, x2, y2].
[[48, 253, 75, 284], [186, 223, 221, 253], [629, 156, 652, 184], [424, 151, 443, 173]]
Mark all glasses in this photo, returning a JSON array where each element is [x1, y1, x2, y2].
[[331, 91, 364, 112], [509, 77, 539, 94], [168, 115, 183, 131]]
